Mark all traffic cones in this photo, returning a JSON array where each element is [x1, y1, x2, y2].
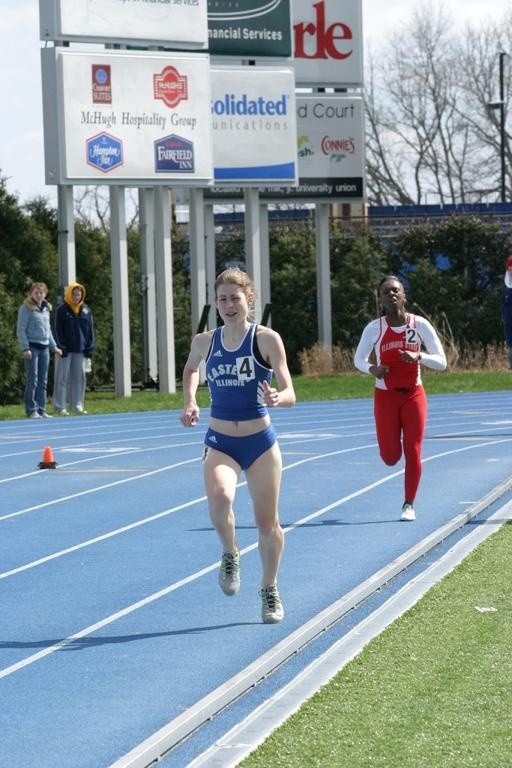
[[38, 446, 57, 470]]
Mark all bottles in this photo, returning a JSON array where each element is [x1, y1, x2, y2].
[[83, 358, 91, 373]]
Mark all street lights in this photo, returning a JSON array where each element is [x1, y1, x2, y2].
[[486, 30, 511, 203]]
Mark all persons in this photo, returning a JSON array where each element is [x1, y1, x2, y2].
[[181, 266, 299, 625], [352, 276, 448, 520], [14, 281, 65, 420], [51, 282, 94, 415], [500, 254, 512, 373]]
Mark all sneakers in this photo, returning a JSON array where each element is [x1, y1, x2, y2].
[[401, 503, 416, 520], [219, 546, 240, 596], [31, 408, 86, 418], [258, 584, 284, 624]]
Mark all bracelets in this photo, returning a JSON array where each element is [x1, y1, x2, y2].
[[418, 352, 423, 360]]
